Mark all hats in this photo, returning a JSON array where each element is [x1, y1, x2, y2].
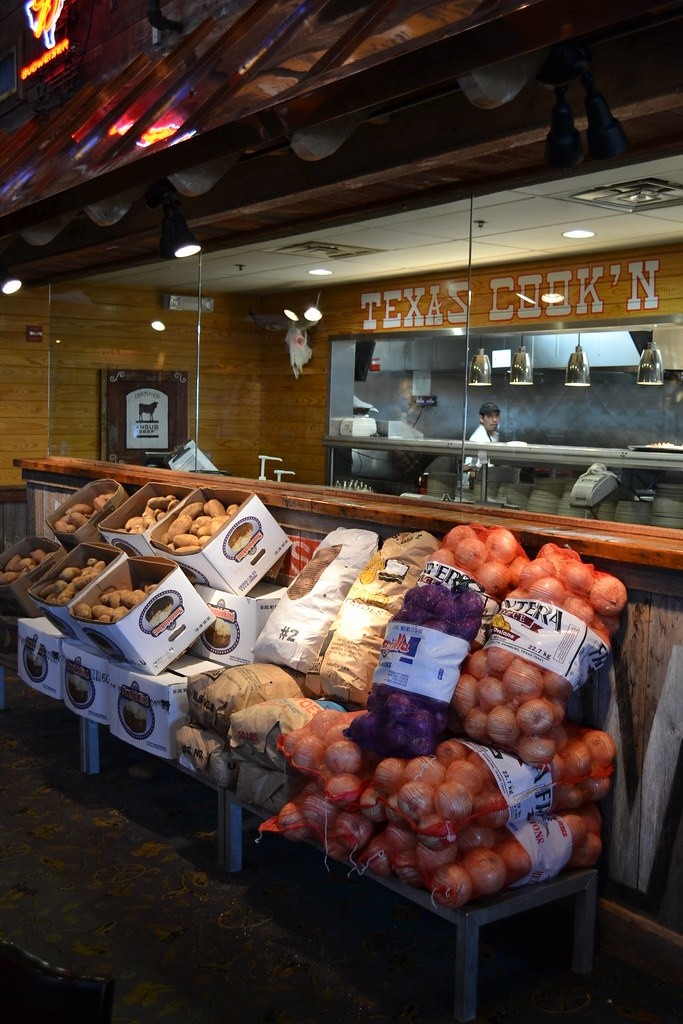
[[480, 402, 500, 414]]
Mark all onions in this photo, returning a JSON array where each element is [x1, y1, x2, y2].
[[276, 525, 626, 908]]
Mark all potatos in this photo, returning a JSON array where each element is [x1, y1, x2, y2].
[[0, 493, 241, 622]]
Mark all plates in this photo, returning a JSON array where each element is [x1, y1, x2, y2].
[[627, 444, 683, 453], [425, 462, 683, 529]]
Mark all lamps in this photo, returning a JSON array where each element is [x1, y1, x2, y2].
[[509, 336, 534, 385], [143, 176, 203, 259], [469, 338, 493, 387], [565, 333, 590, 386], [283, 285, 322, 323], [636, 330, 664, 386], [533, 36, 629, 166]]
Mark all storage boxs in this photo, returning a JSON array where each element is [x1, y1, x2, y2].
[[0, 476, 292, 761]]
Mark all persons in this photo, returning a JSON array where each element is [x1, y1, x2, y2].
[[462, 401, 500, 486]]
